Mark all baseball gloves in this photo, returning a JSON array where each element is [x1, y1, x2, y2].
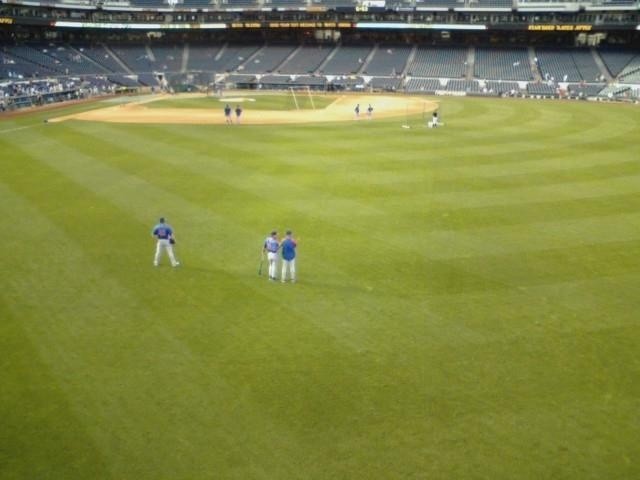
[[170, 238, 176, 246]]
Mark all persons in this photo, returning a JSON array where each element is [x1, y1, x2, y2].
[[354, 103, 361, 120], [279, 229, 298, 285], [149, 215, 181, 269], [432, 109, 439, 129], [222, 103, 233, 127], [0, 70, 120, 111], [259, 229, 280, 282], [233, 104, 243, 125], [366, 103, 374, 119]]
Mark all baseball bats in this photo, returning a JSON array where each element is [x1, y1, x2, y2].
[[258, 256, 266, 276]]
